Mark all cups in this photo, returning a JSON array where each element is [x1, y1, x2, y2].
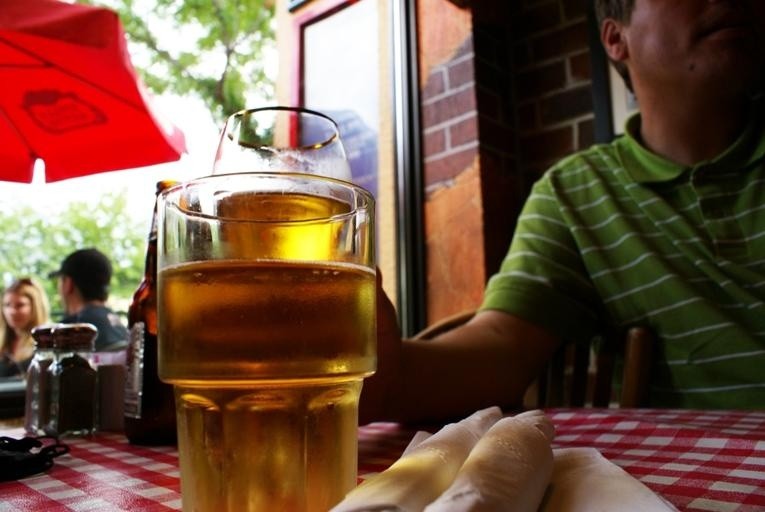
[[212, 106, 356, 184], [94, 364, 127, 435], [155, 171, 379, 512]]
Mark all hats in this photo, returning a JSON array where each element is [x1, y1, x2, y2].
[[49, 248, 111, 284]]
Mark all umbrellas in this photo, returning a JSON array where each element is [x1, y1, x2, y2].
[[0, 0, 180, 187]]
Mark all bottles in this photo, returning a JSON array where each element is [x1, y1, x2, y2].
[[123, 177, 215, 446], [53, 322, 101, 436], [24, 326, 64, 438]]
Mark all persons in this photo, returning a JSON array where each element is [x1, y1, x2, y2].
[[356, 0, 765, 423], [0, 277, 52, 378], [50, 249, 127, 353]]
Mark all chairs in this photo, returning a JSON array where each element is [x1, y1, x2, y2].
[[412, 308, 643, 410]]
